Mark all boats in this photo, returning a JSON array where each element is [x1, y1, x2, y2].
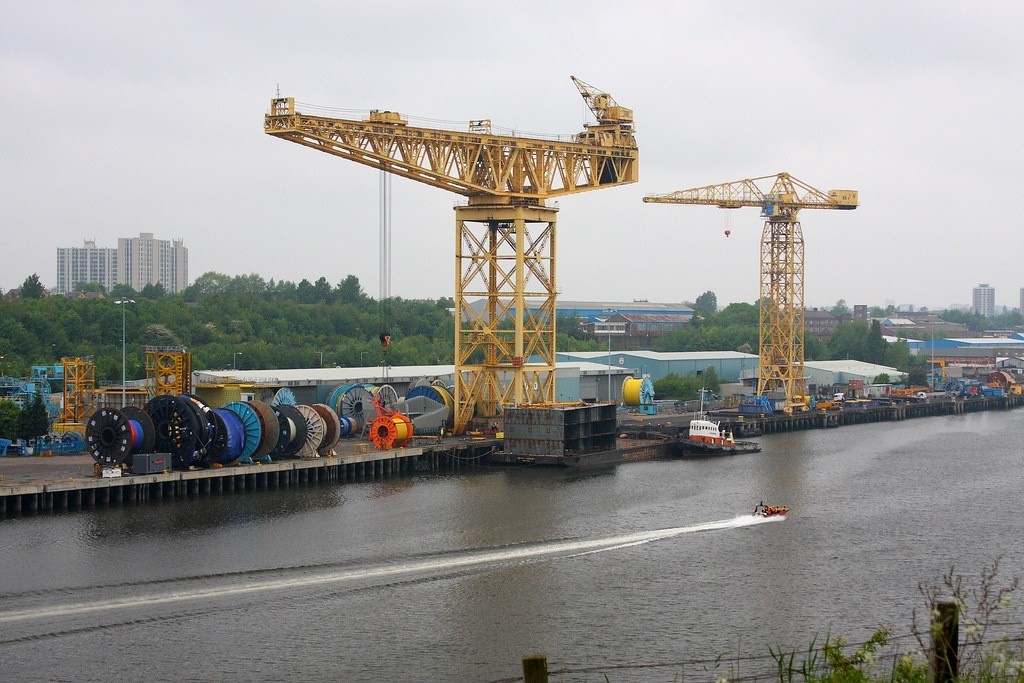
[[679, 386, 762, 458], [753, 500, 790, 517]]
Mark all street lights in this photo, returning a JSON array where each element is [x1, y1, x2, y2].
[[315, 351, 323, 366], [602, 309, 617, 405], [113, 296, 136, 408], [927, 314, 938, 393], [360, 352, 368, 367], [234, 352, 243, 369]]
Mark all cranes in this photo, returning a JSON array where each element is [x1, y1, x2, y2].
[[643, 172, 861, 416], [262, 76, 640, 437]]
